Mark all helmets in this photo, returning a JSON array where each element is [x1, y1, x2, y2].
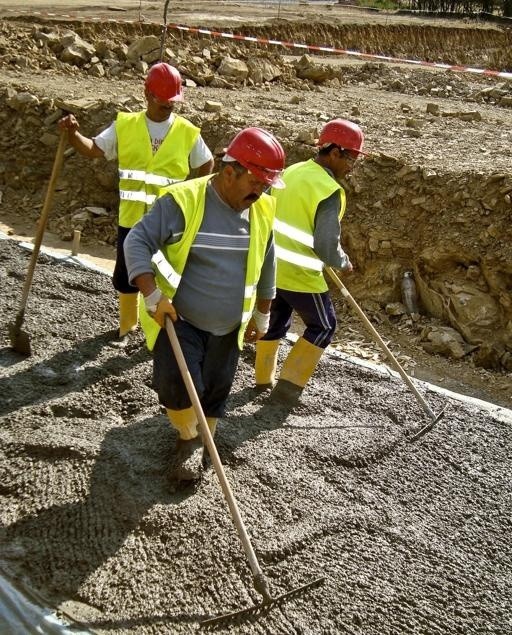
[[316, 118, 370, 157], [144, 63, 184, 102], [223, 127, 286, 189]]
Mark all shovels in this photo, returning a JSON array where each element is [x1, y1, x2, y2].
[[9, 127, 69, 356]]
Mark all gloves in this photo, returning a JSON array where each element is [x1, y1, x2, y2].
[[244, 306, 271, 341], [144, 288, 178, 328]]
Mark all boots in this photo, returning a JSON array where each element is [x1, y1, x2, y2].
[[256, 336, 324, 399], [164, 405, 217, 481], [119, 291, 140, 338]]
